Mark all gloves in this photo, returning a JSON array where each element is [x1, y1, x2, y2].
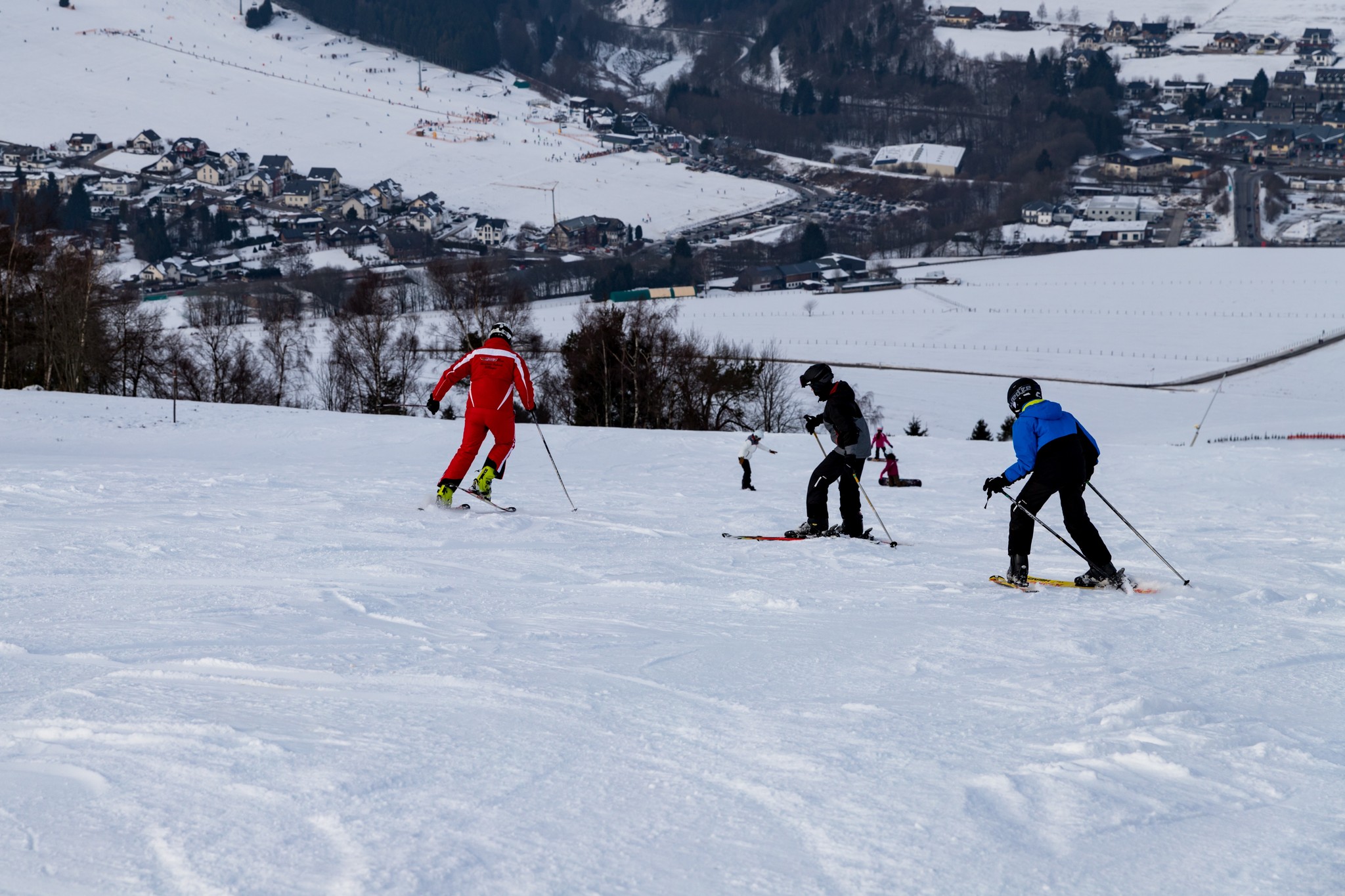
[[770, 450, 778, 454], [740, 457, 744, 465], [871, 446, 873, 449], [526, 402, 537, 412], [983, 472, 1013, 498], [427, 393, 440, 415], [804, 417, 820, 435], [844, 450, 857, 467], [890, 444, 893, 448], [880, 472, 884, 479]]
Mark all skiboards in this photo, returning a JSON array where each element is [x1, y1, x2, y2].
[[722, 528, 881, 545], [417, 483, 517, 513], [988, 570, 1163, 595]]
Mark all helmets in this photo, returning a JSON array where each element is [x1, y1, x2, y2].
[[884, 449, 893, 455], [489, 322, 513, 346], [1007, 378, 1042, 415], [877, 425, 883, 431], [754, 430, 764, 439], [799, 363, 832, 388]]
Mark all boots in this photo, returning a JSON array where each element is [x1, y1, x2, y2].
[[435, 485, 453, 509], [469, 466, 497, 501], [827, 519, 864, 536], [1074, 560, 1118, 587], [1006, 553, 1030, 587], [785, 516, 830, 537]]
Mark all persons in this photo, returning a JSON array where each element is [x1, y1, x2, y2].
[[738, 430, 777, 491], [425, 322, 538, 510], [983, 377, 1125, 586], [871, 425, 892, 459], [880, 448, 907, 487], [784, 363, 871, 539]]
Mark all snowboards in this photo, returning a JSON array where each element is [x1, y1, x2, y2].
[[866, 456, 899, 463], [878, 476, 922, 487]]
